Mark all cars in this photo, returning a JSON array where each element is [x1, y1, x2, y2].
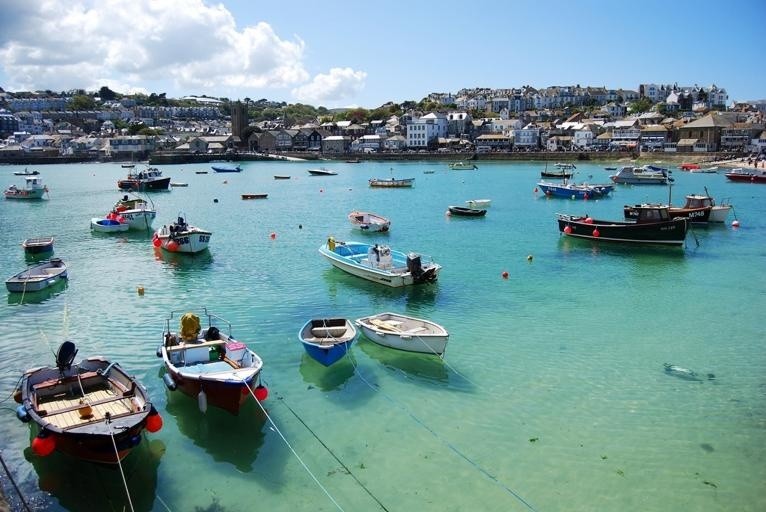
[[362, 143, 543, 154]]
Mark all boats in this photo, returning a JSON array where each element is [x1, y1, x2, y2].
[[356, 312, 449, 358], [154, 220, 210, 254], [5, 169, 67, 291], [212, 161, 487, 231], [297, 316, 356, 370], [159, 307, 264, 417], [317, 238, 441, 292], [91, 163, 208, 234], [16, 341, 153, 463], [541, 161, 765, 245]]
